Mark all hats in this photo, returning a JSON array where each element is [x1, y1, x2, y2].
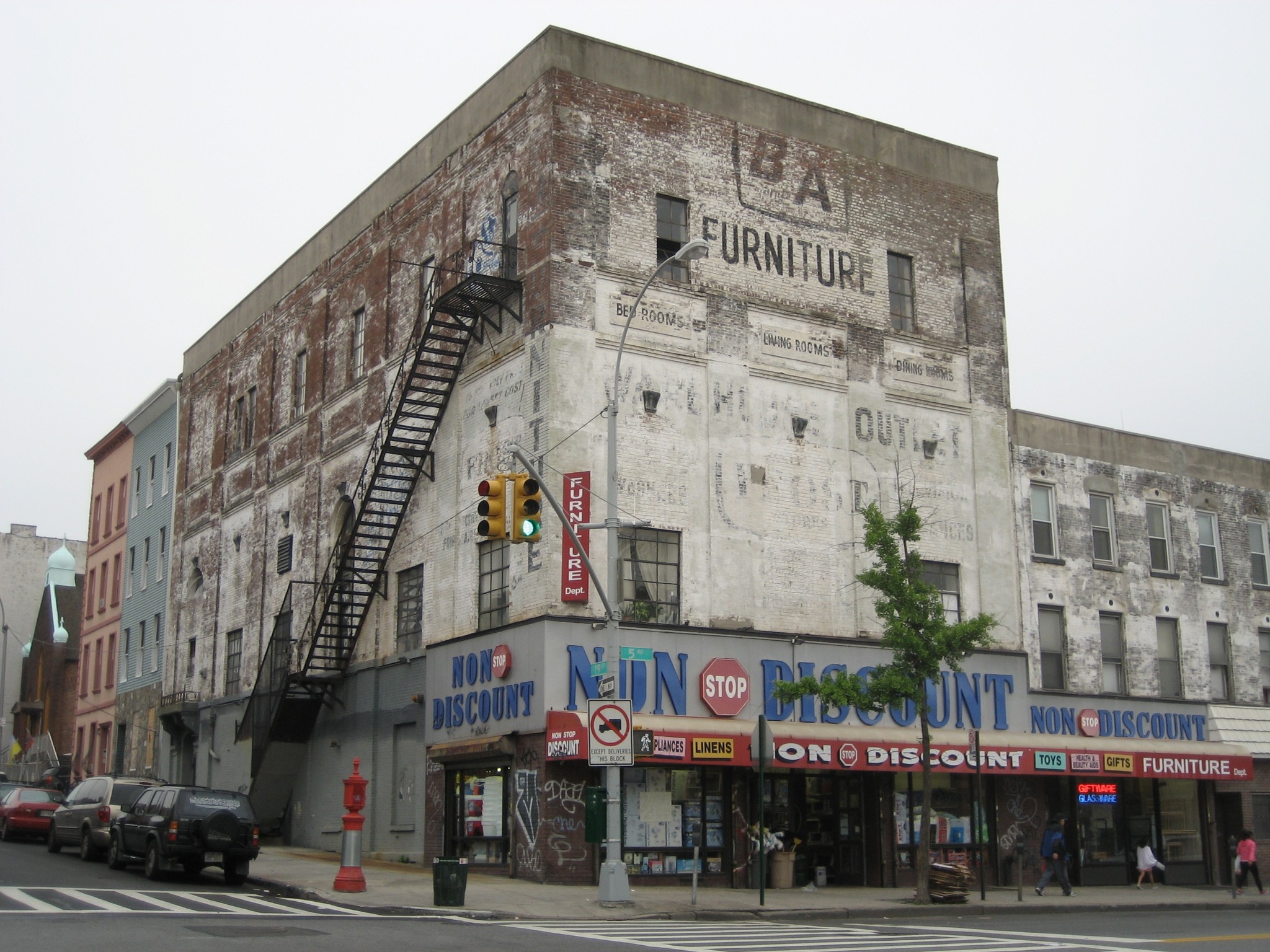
[[1057, 813, 1067, 820]]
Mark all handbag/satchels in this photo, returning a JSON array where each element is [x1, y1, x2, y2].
[[1234, 855, 1241, 875]]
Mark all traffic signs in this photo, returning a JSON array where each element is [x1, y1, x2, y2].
[[597, 674, 616, 698]]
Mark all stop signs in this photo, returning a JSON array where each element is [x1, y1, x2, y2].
[[490, 644, 512, 679], [699, 658, 750, 717], [1079, 708, 1100, 737]]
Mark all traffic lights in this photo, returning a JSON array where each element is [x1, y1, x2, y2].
[[634, 729, 655, 758], [508, 472, 541, 544], [477, 473, 507, 540]]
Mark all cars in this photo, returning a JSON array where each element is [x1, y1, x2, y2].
[[0, 781, 35, 804], [0, 787, 66, 844]]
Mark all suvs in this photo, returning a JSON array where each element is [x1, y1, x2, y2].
[[46, 773, 165, 862], [108, 783, 261, 887]]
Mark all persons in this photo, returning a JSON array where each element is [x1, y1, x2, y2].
[[1135, 836, 1158, 890], [1234, 830, 1267, 895], [1035, 814, 1076, 896]]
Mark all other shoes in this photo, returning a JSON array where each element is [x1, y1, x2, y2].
[[1151, 886, 1157, 889], [1260, 889, 1269, 896], [1137, 885, 1142, 890], [1063, 892, 1077, 896], [1236, 890, 1244, 896], [1036, 888, 1044, 895]]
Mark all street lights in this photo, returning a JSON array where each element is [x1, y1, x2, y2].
[[597, 238, 712, 907]]
